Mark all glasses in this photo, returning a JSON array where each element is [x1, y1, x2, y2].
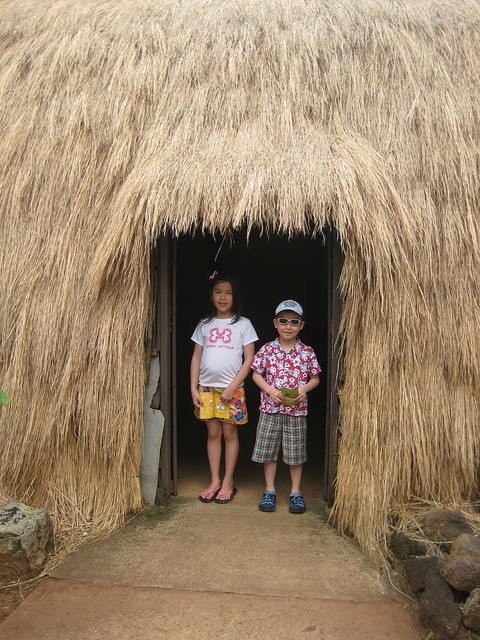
[[276, 316, 302, 326]]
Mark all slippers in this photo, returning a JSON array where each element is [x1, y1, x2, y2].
[[199, 483, 222, 503], [215, 486, 237, 504]]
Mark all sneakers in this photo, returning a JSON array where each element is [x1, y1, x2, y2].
[[259, 491, 278, 511], [288, 493, 307, 513]]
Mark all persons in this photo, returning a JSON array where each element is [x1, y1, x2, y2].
[[190, 271, 259, 505], [251, 300, 321, 513]]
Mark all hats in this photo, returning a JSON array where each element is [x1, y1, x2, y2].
[[274, 299, 304, 316]]
